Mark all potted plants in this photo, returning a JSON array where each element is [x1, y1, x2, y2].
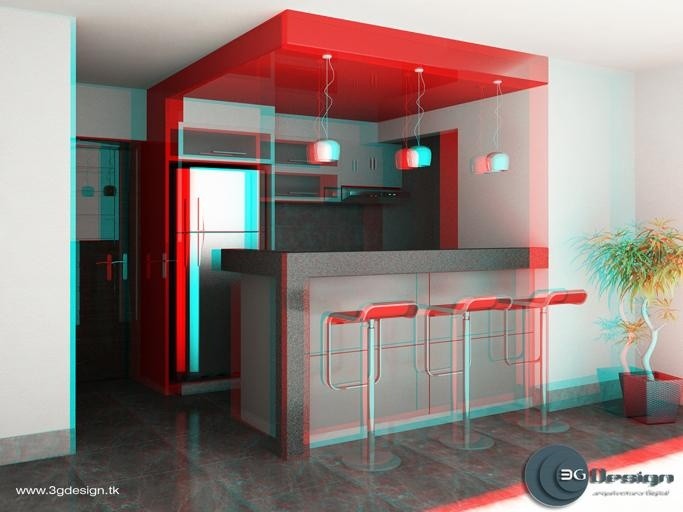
[[568, 217, 683, 426]]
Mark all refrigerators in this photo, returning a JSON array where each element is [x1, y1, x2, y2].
[[173, 167, 260, 378]]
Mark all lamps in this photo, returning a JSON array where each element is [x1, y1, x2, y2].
[[484, 78, 510, 173], [312, 53, 341, 163], [406, 66, 432, 167]]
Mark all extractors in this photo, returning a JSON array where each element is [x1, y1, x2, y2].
[[340, 186, 404, 207]]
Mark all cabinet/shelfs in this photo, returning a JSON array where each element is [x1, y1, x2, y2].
[[75, 140, 119, 243]]
